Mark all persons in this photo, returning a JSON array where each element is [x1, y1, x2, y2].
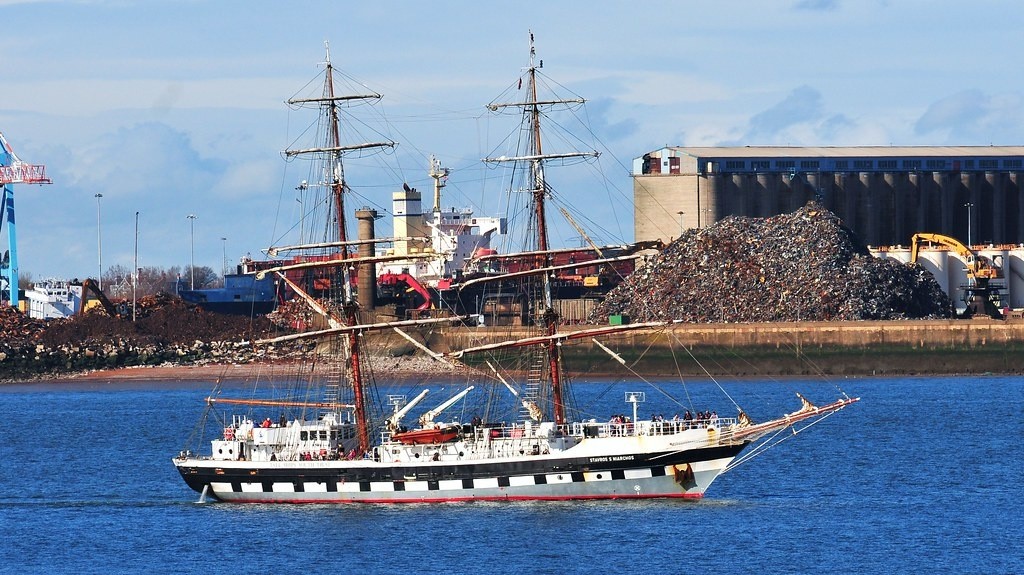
[[251, 413, 287, 428], [651, 409, 720, 436], [472, 415, 482, 438], [609, 414, 628, 436], [393, 424, 409, 435], [298, 441, 380, 464]]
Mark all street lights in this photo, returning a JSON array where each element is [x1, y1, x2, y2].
[[186, 215, 197, 292], [94, 191, 105, 290]]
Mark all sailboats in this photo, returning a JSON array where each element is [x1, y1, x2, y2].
[[172, 30, 861, 508]]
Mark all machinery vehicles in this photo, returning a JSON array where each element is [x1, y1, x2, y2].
[[77, 278, 130, 323], [907, 233, 1009, 319]]
